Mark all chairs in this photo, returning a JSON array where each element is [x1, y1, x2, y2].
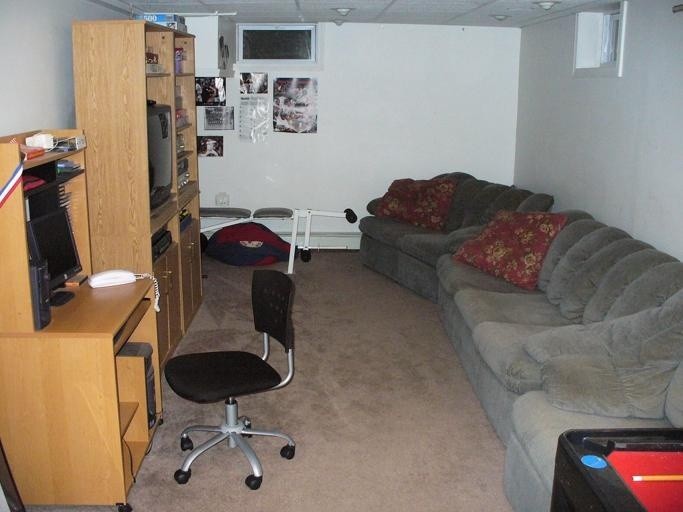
[[164, 270, 297, 490]]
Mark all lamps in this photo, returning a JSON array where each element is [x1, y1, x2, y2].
[[334, 19, 342, 25], [335, 8, 354, 15], [538, 3, 554, 9], [496, 15, 505, 21]]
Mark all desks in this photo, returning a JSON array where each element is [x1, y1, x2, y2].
[[550, 427, 682, 512]]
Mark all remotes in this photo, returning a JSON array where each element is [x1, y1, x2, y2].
[[146, 96, 158, 104]]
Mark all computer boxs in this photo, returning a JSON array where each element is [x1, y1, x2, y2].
[[114, 341, 158, 429]]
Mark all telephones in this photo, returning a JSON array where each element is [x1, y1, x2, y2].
[[87, 269, 138, 290]]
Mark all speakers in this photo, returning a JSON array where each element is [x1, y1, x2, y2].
[[28, 261, 53, 330]]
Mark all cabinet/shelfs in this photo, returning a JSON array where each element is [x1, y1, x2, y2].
[[0, 129, 163, 512], [72, 17, 203, 365]]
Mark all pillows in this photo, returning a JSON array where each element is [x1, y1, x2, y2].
[[377, 178, 456, 230], [452, 208, 569, 293]]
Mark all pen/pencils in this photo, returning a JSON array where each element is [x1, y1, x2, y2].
[[632, 475, 683, 481]]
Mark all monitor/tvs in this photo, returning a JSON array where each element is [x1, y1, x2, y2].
[[149, 107, 174, 209], [28, 206, 85, 307]]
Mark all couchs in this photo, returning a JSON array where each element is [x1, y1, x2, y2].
[[435, 209, 683, 512], [360, 172, 555, 303]]
